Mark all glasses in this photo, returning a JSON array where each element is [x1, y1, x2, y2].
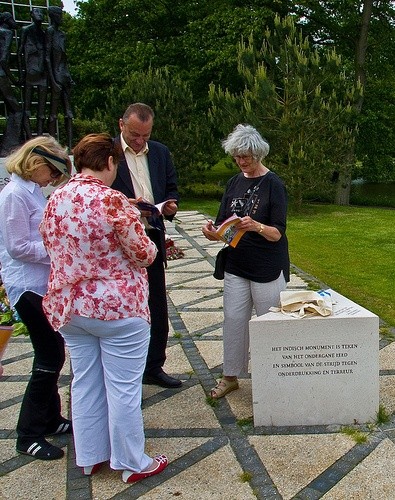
[[45, 163, 62, 178], [232, 155, 250, 159]]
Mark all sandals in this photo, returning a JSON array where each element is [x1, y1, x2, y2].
[[208, 377, 240, 400]]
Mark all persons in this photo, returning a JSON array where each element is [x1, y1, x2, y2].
[[202, 124, 291, 397], [0, 12, 23, 114], [0, 138, 73, 461], [18, 8, 48, 139], [45, 7, 75, 155], [42, 134, 169, 483], [111, 104, 181, 389]]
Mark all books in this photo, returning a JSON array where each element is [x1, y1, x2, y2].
[[136, 196, 177, 217], [209, 215, 247, 248]]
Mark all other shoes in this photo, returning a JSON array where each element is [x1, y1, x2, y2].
[[16, 436, 65, 460], [81, 462, 99, 474], [15, 418, 73, 435], [141, 367, 183, 389], [121, 453, 168, 484]]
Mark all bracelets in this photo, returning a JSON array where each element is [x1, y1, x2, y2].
[[258, 224, 263, 233]]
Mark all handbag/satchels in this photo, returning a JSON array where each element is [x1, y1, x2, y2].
[[269, 288, 333, 318]]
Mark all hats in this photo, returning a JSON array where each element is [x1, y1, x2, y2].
[[33, 149, 71, 179]]
